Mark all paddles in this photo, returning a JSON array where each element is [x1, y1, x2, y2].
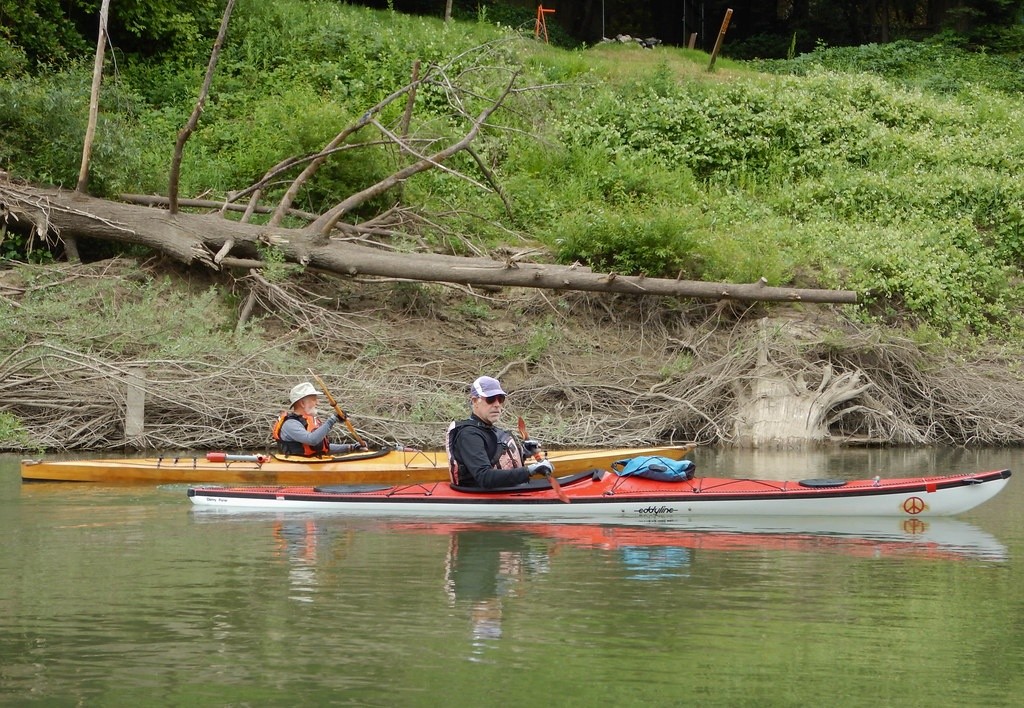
[[517, 416, 568, 506], [306, 367, 366, 449]]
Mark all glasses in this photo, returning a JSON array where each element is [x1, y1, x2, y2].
[[476, 394, 505, 405]]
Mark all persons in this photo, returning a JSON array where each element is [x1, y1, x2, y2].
[[273, 519, 349, 591], [442, 529, 552, 637], [274, 382, 369, 458], [446, 376, 555, 488]]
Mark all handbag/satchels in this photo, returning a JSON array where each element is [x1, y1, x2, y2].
[[611, 456, 696, 481]]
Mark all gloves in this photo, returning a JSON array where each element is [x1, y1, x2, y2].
[[354, 440, 369, 451], [521, 440, 542, 458], [335, 410, 347, 422], [528, 459, 554, 476]]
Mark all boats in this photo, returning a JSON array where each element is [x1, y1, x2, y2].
[[19, 443, 699, 485], [187, 466, 1014, 526]]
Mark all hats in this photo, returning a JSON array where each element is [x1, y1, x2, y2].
[[289, 382, 323, 409], [471, 376, 507, 398]]
